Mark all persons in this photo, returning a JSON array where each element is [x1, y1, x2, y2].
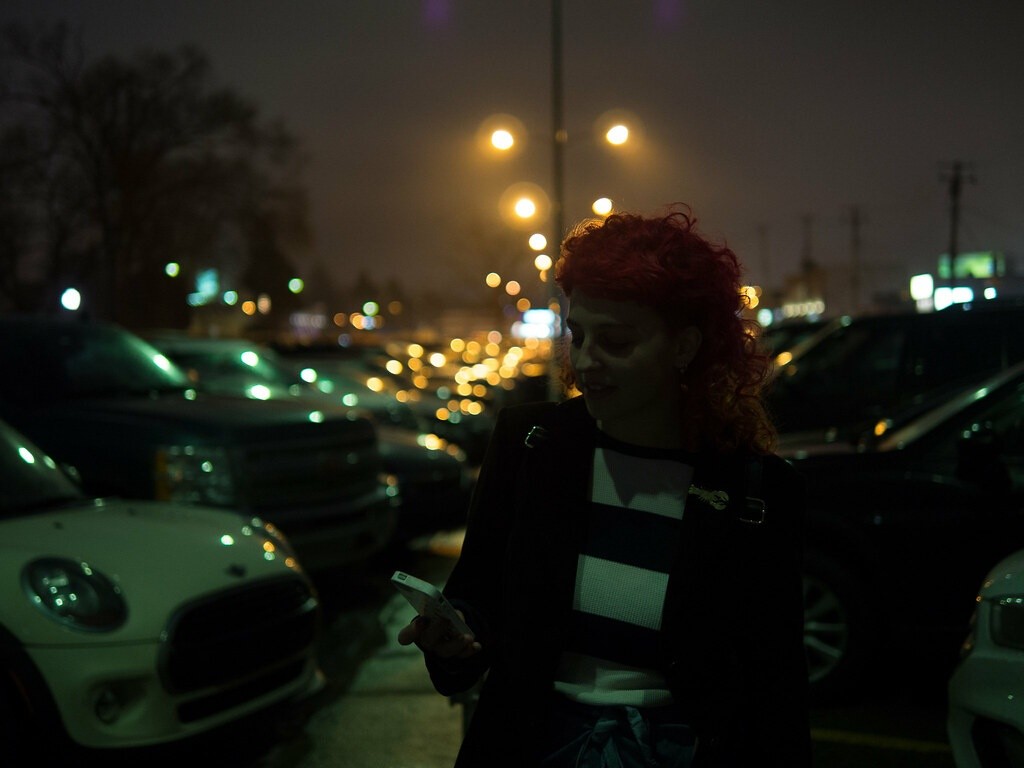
[[392, 212, 876, 768]]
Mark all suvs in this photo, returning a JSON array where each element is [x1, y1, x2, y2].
[[1, 313, 400, 599]]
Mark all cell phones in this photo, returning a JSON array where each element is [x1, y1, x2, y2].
[[391, 571, 475, 642]]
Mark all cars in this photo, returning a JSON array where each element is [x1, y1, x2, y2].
[[150, 321, 554, 550], [1, 420, 329, 768], [751, 298, 1024, 768]]
